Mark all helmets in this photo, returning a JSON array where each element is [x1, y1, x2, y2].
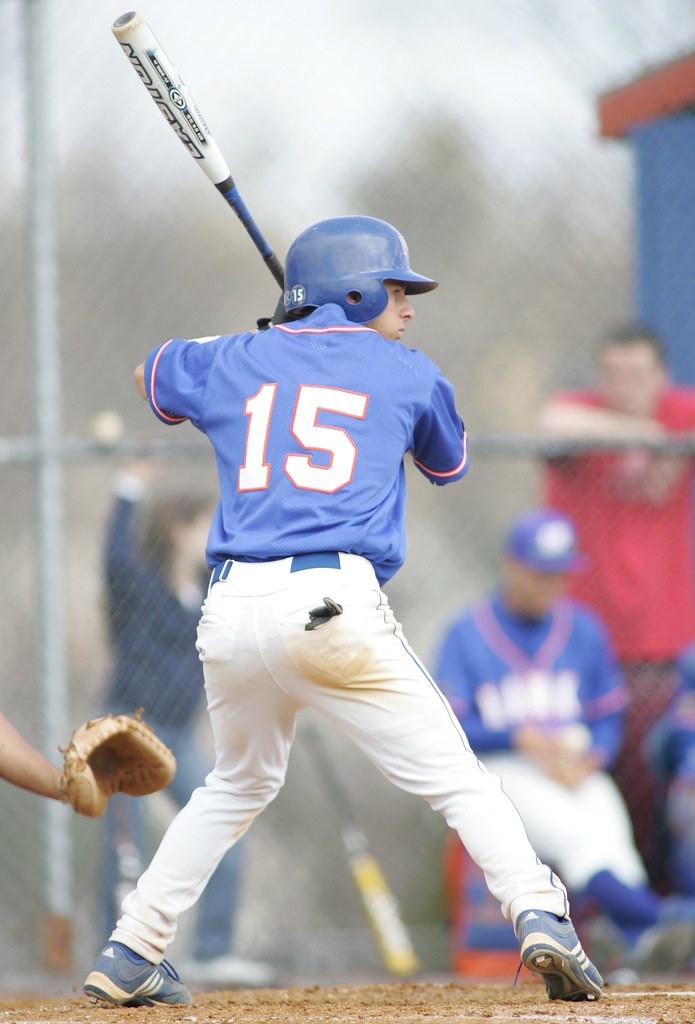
[[282, 216, 438, 323]]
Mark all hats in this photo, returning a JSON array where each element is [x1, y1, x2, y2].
[[506, 511, 587, 578]]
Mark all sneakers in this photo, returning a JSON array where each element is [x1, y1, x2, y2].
[[83, 942, 193, 1009], [513, 911, 603, 1001]]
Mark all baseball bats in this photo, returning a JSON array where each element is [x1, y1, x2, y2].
[[302, 721, 417, 977], [110, 9, 285, 290]]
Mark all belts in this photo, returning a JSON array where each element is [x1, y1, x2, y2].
[[209, 548, 341, 589]]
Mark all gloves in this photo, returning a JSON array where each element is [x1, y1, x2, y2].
[[304, 595, 343, 632]]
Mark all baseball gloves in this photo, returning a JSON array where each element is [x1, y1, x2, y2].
[[58, 711, 179, 819]]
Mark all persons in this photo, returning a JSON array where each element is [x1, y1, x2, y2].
[[0, 708, 178, 819], [82, 214, 695, 1008]]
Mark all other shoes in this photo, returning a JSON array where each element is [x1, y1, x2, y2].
[[635, 895, 695, 976], [202, 958, 280, 989]]
